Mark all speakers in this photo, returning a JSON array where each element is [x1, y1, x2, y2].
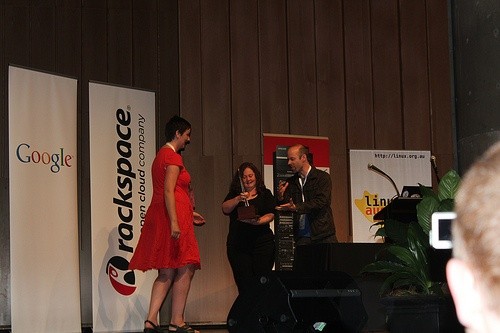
[[227, 271, 367, 333]]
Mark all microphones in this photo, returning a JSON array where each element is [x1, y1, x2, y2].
[[369, 163, 400, 197], [431, 155, 440, 184]]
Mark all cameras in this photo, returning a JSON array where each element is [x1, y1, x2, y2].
[[428, 211, 458, 250]]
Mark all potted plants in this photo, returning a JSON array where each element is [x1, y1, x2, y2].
[[359, 170, 464, 333]]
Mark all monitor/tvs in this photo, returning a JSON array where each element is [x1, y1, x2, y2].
[[401, 186, 432, 198]]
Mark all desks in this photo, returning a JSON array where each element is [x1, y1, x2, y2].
[[279, 243, 386, 333]]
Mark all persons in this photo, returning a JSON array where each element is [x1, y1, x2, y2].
[[220, 163, 294, 333], [128, 113, 207, 333], [445, 142, 500, 333], [274, 144, 344, 243]]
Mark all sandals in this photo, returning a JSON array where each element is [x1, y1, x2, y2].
[[169, 323, 199, 333], [143, 320, 168, 333]]
